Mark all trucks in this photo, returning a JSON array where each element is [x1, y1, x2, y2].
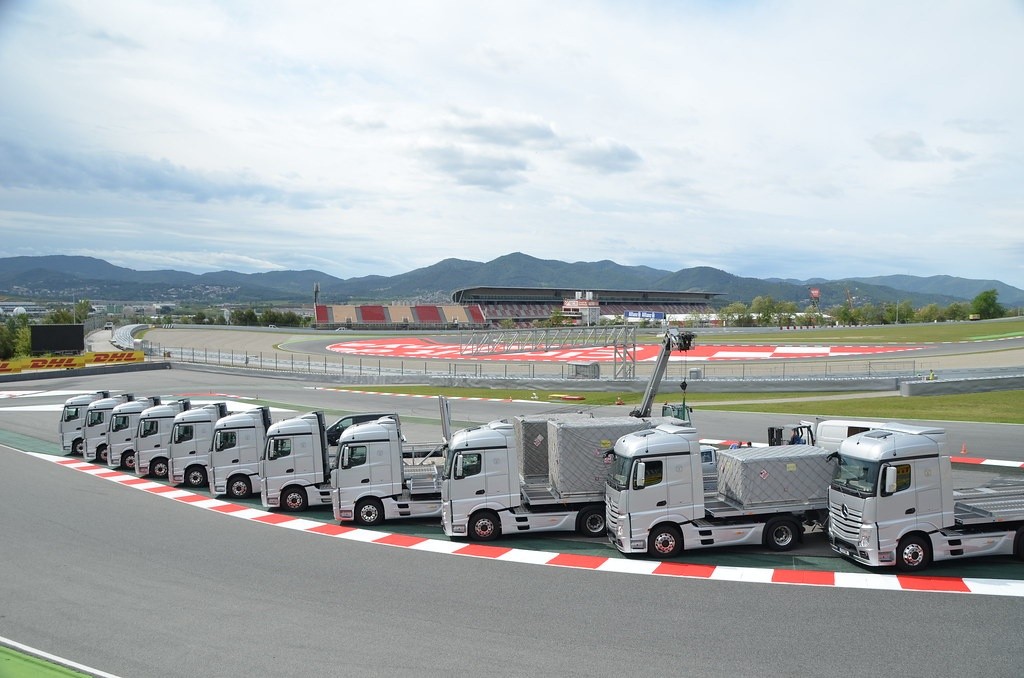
[[441, 417, 607, 542], [104, 321, 113, 330], [57, 389, 266, 502], [259, 409, 335, 513], [325, 412, 447, 456], [331, 411, 445, 527], [802, 419, 887, 450], [825, 426, 1024, 573], [603, 426, 838, 560]]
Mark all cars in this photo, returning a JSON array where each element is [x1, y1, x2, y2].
[[700, 448, 718, 474]]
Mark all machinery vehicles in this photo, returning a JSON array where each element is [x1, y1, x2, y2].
[[710, 426, 816, 449], [619, 324, 694, 423]]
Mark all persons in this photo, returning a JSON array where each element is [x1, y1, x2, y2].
[[729, 440, 742, 449], [929, 369, 934, 380], [617, 397, 623, 405], [746, 442, 752, 448]]
